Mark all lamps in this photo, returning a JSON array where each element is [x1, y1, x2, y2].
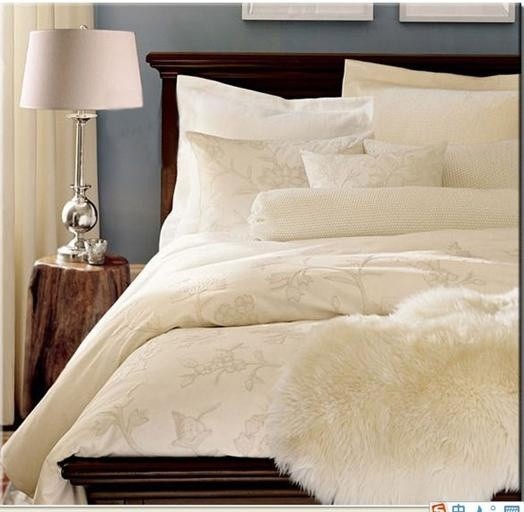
[[18, 26, 144, 264]]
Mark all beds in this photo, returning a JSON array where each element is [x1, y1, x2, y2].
[[57, 51, 519, 504]]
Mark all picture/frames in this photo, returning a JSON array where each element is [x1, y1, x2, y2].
[[399, 3, 516, 22], [242, 3, 374, 21]]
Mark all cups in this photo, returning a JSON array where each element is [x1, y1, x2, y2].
[[84, 239, 108, 265]]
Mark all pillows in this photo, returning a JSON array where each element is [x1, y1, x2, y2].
[[363, 137, 518, 190], [299, 141, 447, 186], [159, 74, 373, 249], [245, 186, 519, 241], [341, 59, 518, 144], [186, 130, 375, 237]]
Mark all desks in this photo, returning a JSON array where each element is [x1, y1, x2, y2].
[[23, 254, 130, 418]]
